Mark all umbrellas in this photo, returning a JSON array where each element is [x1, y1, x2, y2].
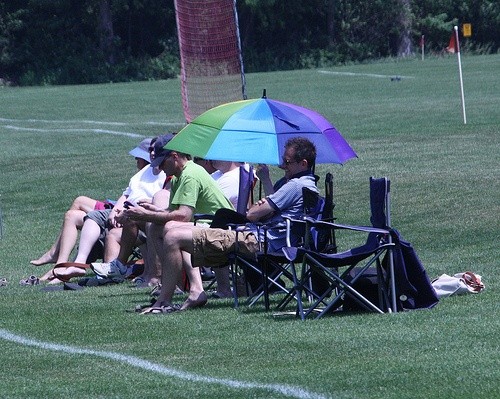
[[163, 88, 360, 200]]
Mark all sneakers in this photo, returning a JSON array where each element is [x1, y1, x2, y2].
[[89, 260, 126, 282]]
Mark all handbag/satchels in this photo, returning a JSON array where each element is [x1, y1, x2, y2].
[[432, 270, 484, 295]]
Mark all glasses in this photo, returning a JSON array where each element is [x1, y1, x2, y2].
[[282, 157, 301, 164], [148, 146, 154, 152]]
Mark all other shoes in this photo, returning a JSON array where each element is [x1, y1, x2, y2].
[[20, 276, 40, 285], [0, 278, 7, 286]]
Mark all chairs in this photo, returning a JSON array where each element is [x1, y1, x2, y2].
[[193, 164, 397, 322]]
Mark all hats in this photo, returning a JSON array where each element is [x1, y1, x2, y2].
[[150, 134, 174, 167], [129, 139, 152, 163]]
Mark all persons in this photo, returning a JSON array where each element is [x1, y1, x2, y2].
[[138, 138, 320, 315], [30, 133, 250, 296]]
